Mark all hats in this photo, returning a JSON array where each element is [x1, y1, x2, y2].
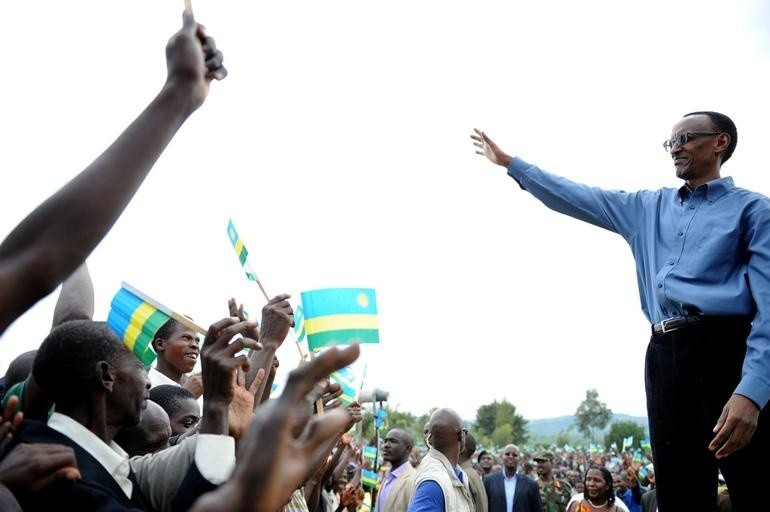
[[534, 451, 554, 462], [477, 449, 490, 462]]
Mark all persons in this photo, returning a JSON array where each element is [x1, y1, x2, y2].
[[1, 261, 372, 512], [0, 0, 229, 336], [470, 110, 770, 512], [373, 406, 732, 512]]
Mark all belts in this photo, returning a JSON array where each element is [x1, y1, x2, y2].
[[651, 314, 706, 334]]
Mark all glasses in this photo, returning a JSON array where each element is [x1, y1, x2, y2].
[[663, 129, 720, 151], [505, 452, 518, 457]]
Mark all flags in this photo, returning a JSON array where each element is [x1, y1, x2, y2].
[[224, 220, 265, 285], [300, 288, 381, 351]]
